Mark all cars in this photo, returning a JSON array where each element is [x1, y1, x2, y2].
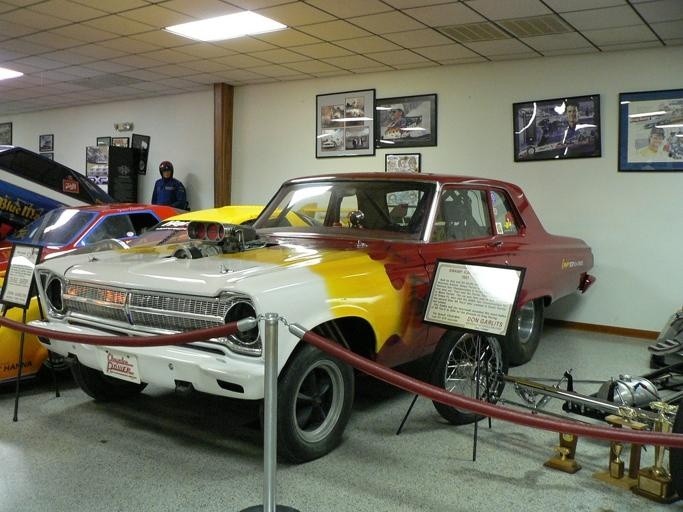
[[33, 145, 595, 465], [2, 144, 355, 387]]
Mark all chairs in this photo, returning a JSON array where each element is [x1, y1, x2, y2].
[[435, 200, 469, 227]]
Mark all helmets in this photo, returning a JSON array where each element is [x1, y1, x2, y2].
[[159, 161, 174, 179]]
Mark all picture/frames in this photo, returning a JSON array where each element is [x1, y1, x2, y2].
[[0, 123, 12, 145], [315, 88, 437, 207], [37, 135, 53, 162], [419, 255, 527, 339], [96, 133, 151, 177], [510, 89, 683, 174]]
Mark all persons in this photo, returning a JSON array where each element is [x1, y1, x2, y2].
[[149, 160, 187, 210], [408, 191, 417, 203], [546, 102, 597, 160], [387, 155, 397, 169], [407, 157, 418, 172], [637, 126, 665, 158], [397, 155, 408, 168], [387, 194, 396, 204], [383, 104, 410, 137], [666, 130, 676, 144]]
